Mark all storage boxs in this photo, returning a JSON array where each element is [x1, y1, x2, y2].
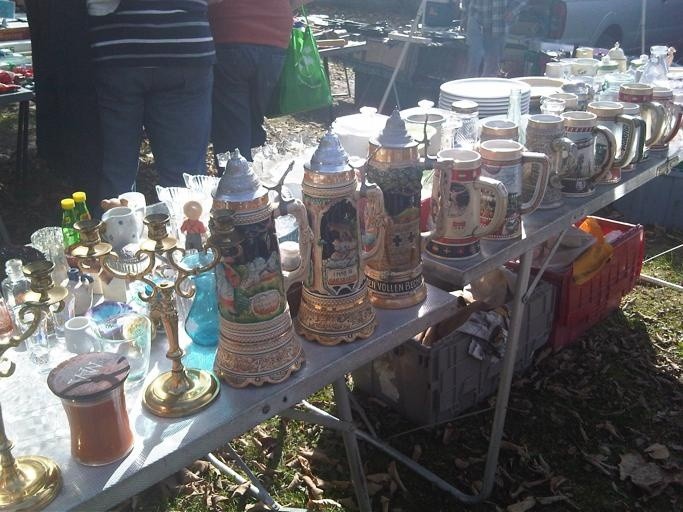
[[350, 264, 557, 435], [506, 215, 644, 355]]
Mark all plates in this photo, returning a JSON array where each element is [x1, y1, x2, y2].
[[438, 77, 531, 121], [512, 75, 567, 115], [88, 300, 137, 340]]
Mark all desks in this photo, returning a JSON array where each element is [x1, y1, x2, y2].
[[1, 259, 466, 511], [281, 130, 682, 510], [0, 73, 34, 191], [318, 33, 368, 102]]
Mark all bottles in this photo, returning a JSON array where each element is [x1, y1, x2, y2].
[[296, 126, 387, 348], [80, 259, 127, 303], [0, 259, 32, 338], [95, 191, 146, 261], [60, 268, 94, 316], [650, 45, 676, 79], [180, 253, 220, 347], [361, 110, 453, 313], [423, 99, 550, 261], [208, 145, 315, 392], [144, 277, 178, 334], [59, 192, 94, 248]]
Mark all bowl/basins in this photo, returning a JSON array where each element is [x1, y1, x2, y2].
[[572, 57, 600, 77]]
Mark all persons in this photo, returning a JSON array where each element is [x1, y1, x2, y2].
[[208, 0, 312, 177], [464, 0, 530, 76]]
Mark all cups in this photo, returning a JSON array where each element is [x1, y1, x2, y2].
[[619, 83, 683, 171], [576, 47, 593, 58], [598, 41, 651, 77], [154, 171, 223, 245], [95, 313, 151, 382], [30, 225, 66, 270], [545, 62, 563, 78], [523, 101, 639, 208], [17, 309, 51, 365], [47, 352, 136, 468], [49, 291, 100, 354]]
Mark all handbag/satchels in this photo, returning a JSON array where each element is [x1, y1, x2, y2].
[[278, 20, 332, 114]]
